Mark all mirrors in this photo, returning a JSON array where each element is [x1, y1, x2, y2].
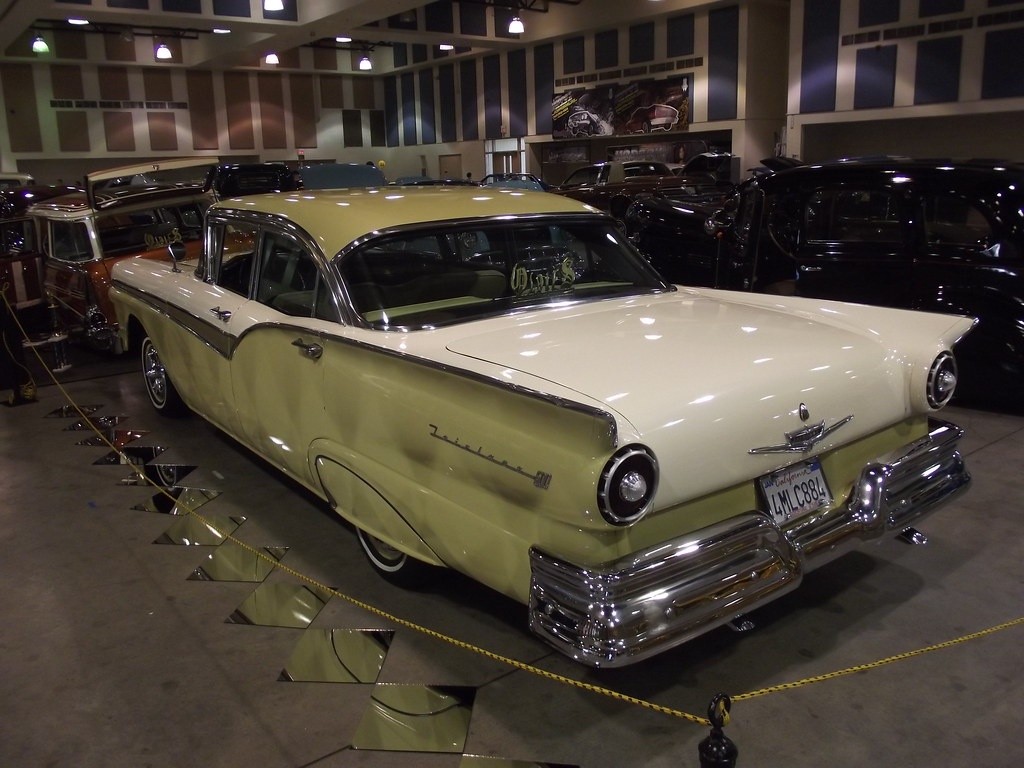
[[41, 403, 103, 418], [350, 682, 476, 752], [115, 463, 199, 488], [185, 544, 293, 583], [224, 578, 339, 629], [73, 427, 154, 450], [63, 415, 129, 431], [458, 753, 582, 768], [274, 627, 395, 684], [149, 510, 249, 545], [91, 447, 168, 465], [131, 486, 223, 515]]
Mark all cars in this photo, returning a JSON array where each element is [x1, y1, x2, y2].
[[285, 164, 389, 186], [212, 161, 301, 198], [481, 173, 556, 194], [112, 186, 983, 675], [624, 158, 1024, 413], [22, 157, 261, 358], [550, 160, 684, 216], [0, 185, 90, 321]]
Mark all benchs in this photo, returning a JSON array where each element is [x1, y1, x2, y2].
[[270, 271, 508, 318]]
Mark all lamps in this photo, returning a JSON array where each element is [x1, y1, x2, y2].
[[156, 41, 172, 59], [509, 10, 524, 33], [360, 51, 372, 70]]
